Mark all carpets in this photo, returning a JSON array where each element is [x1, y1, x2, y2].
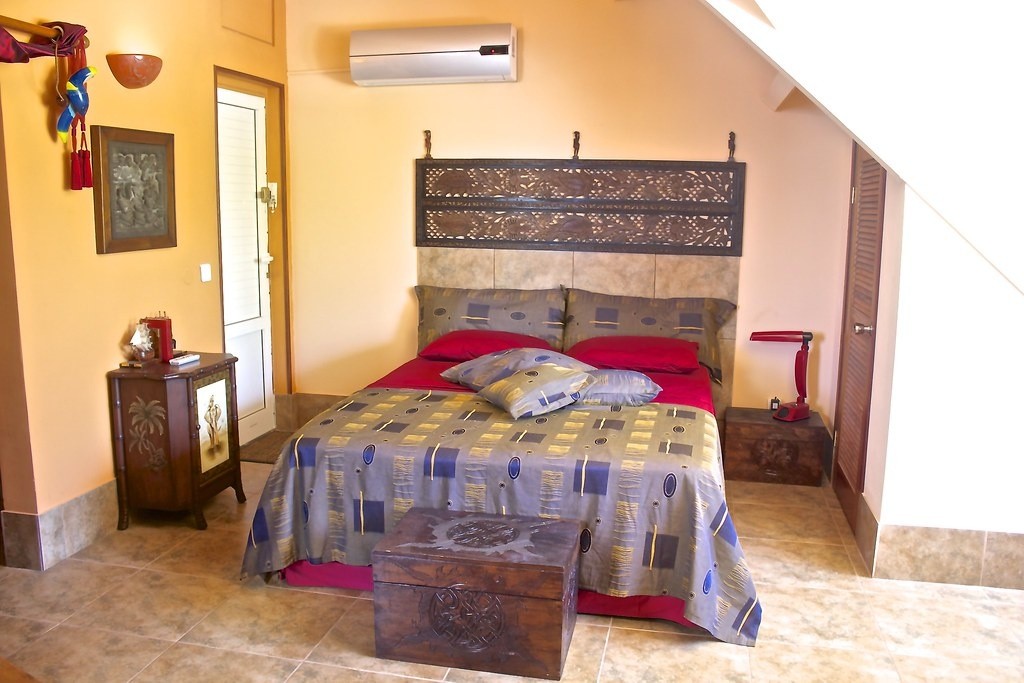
[[240, 429, 296, 464]]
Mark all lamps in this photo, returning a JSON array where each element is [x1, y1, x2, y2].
[[749, 330, 814, 423]]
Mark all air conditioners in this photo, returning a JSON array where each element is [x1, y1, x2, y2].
[[348, 22, 520, 87]]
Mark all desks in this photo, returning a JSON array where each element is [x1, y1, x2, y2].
[[105, 350, 247, 530]]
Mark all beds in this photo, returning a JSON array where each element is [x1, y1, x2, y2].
[[240, 246, 764, 649]]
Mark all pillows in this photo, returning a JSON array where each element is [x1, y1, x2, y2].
[[417, 330, 561, 362], [559, 284, 738, 388], [413, 285, 565, 354], [564, 335, 702, 375], [438, 347, 598, 393], [572, 370, 664, 407], [475, 362, 590, 421]]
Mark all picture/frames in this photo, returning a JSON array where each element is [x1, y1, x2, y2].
[[89, 124, 178, 256]]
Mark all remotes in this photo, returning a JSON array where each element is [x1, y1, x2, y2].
[[169, 354, 200, 366]]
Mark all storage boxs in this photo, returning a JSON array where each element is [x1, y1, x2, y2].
[[370, 505, 587, 680], [724, 405, 826, 487]]
